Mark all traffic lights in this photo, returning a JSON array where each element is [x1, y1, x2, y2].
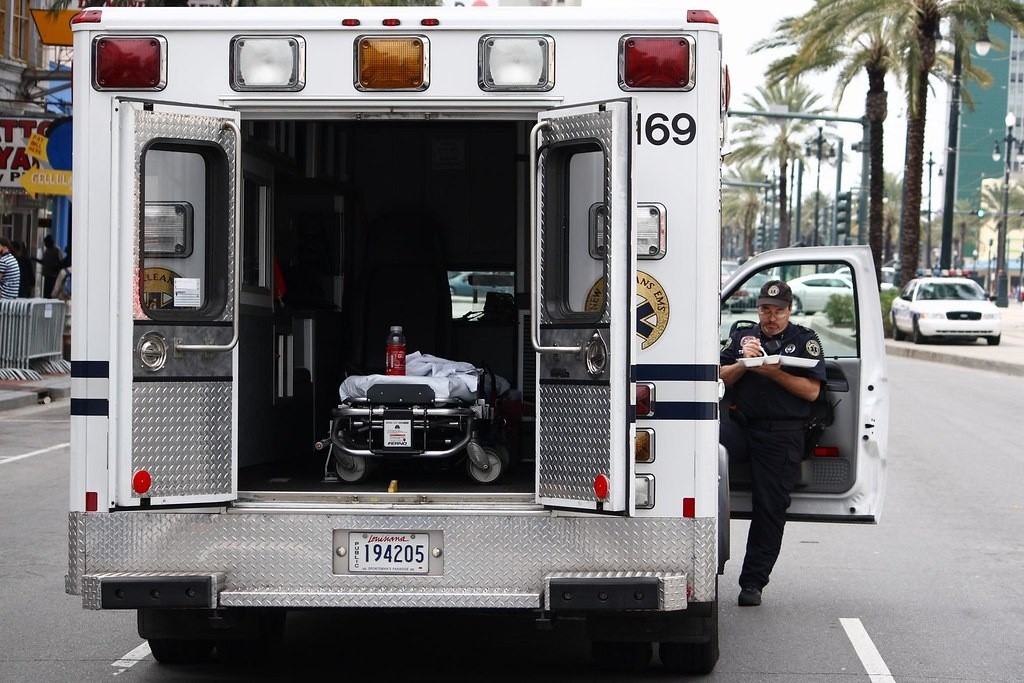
[[834, 189, 852, 236]]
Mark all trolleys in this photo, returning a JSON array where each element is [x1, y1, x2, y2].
[[322, 373, 513, 487]]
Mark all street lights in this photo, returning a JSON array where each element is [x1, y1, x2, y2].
[[993, 110, 1024, 308], [802, 122, 836, 251], [923, 149, 945, 271]]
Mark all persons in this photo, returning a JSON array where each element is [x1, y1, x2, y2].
[[0, 234, 71, 360], [720, 281, 826, 606]]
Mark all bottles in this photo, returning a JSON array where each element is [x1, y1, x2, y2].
[[386, 326, 406, 376]]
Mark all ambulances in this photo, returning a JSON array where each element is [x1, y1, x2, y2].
[[63, 2, 888, 683]]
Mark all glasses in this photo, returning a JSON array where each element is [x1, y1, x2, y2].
[[758, 309, 787, 321]]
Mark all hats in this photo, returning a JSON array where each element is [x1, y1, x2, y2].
[[755, 280, 793, 308]]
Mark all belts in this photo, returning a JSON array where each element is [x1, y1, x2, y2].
[[733, 418, 807, 431]]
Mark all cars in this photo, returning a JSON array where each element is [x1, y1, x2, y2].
[[719, 258, 772, 315], [833, 266, 897, 293], [785, 273, 854, 317], [890, 269, 1001, 346]]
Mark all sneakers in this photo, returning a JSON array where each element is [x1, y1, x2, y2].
[[737, 586, 762, 607]]
